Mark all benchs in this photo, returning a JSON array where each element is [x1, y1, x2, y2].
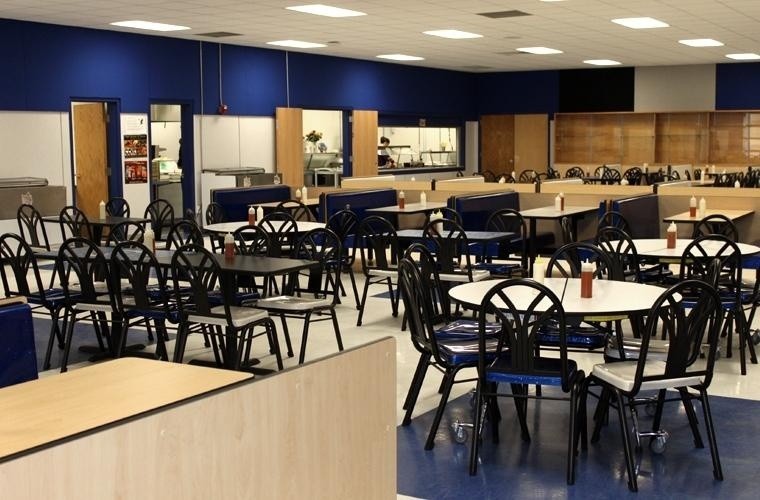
[[208, 180, 661, 238]]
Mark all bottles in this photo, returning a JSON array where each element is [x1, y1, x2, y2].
[[274, 175, 280, 185], [690, 194, 706, 219], [580, 258, 593, 298], [533, 254, 544, 294], [411, 176, 415, 181], [225, 231, 234, 258], [143, 223, 156, 256], [248, 204, 256, 226], [295, 188, 301, 203], [420, 190, 426, 208], [554, 192, 564, 212], [667, 219, 678, 249], [99, 199, 106, 219], [301, 185, 307, 201], [398, 190, 405, 209], [255, 205, 265, 227], [244, 177, 250, 189], [500, 156, 741, 188]]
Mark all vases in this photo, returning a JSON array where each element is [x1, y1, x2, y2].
[[310, 142, 318, 153]]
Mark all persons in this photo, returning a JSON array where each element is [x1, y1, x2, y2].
[[378, 136, 394, 169]]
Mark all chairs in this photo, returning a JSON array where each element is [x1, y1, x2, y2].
[[0, 197, 403, 373], [466, 276, 590, 486], [403, 204, 760, 376], [593, 280, 726, 493], [455, 163, 757, 192], [396, 244, 533, 456]]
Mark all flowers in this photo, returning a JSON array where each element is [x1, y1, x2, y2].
[[305, 130, 323, 143]]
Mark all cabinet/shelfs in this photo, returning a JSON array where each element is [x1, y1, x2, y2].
[[552, 111, 758, 166]]
